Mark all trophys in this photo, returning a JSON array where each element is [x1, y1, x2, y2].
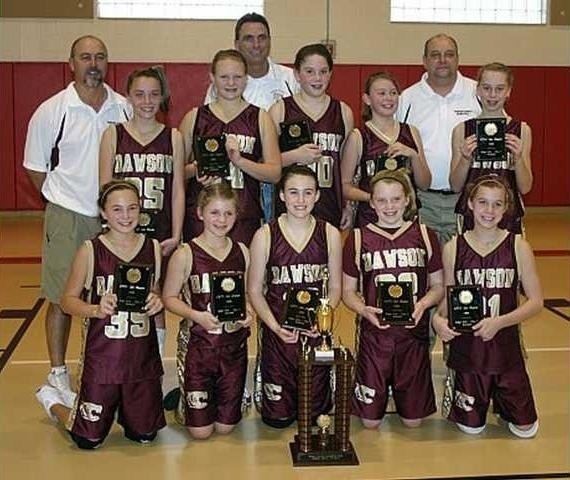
[[309, 265, 339, 359]]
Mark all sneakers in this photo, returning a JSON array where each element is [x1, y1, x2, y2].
[[34, 386, 71, 422], [164, 388, 181, 412], [46, 369, 73, 393]]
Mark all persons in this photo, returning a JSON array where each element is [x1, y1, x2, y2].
[[175, 49, 282, 249], [431, 165, 548, 439], [18, 32, 131, 388], [390, 34, 487, 352], [94, 58, 186, 359], [449, 61, 535, 239], [161, 185, 252, 438], [206, 11, 307, 227], [337, 167, 444, 428], [266, 42, 358, 237], [244, 161, 348, 431], [340, 69, 431, 228], [31, 177, 165, 451]]
